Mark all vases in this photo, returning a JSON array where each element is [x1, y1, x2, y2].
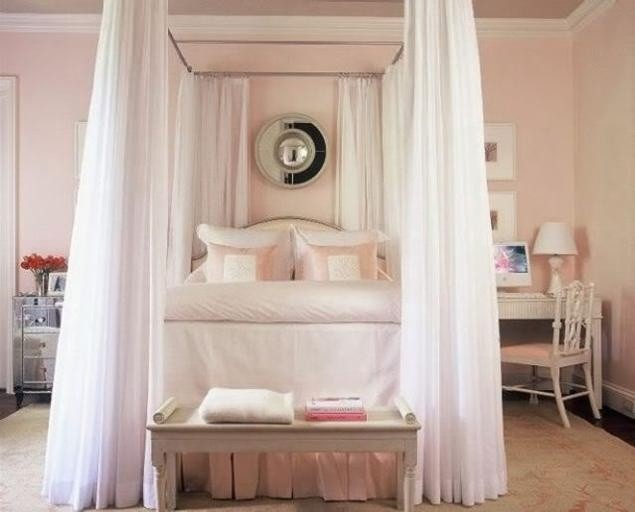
[[35, 275, 48, 296]]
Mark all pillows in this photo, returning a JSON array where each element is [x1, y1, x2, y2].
[[291, 223, 378, 281], [302, 242, 378, 281], [206, 242, 279, 281], [197, 223, 293, 281]]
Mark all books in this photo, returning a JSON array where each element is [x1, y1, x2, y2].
[[304, 396, 367, 421]]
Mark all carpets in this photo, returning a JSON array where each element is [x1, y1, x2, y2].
[[1, 402, 634, 512]]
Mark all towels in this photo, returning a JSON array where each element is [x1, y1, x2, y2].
[[202, 387, 293, 424]]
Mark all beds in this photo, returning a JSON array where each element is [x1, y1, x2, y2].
[[163, 215, 403, 500]]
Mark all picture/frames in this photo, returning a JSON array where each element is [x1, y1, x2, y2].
[[484, 122, 518, 182], [12, 296, 55, 327], [488, 189, 517, 244], [47, 272, 66, 296], [21, 305, 57, 330], [75, 122, 87, 179]]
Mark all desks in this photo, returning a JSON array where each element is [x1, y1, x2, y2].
[[496, 289, 605, 410], [148, 406, 422, 512]]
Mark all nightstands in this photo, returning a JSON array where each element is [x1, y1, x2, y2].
[[12, 327, 64, 406]]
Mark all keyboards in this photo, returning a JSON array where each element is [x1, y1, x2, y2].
[[497, 292, 547, 299]]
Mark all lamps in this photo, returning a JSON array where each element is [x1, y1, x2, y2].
[[532, 222, 578, 298]]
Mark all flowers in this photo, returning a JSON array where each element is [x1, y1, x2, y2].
[[21, 254, 66, 281]]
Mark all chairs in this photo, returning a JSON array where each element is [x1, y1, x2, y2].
[[500, 280, 601, 428]]
